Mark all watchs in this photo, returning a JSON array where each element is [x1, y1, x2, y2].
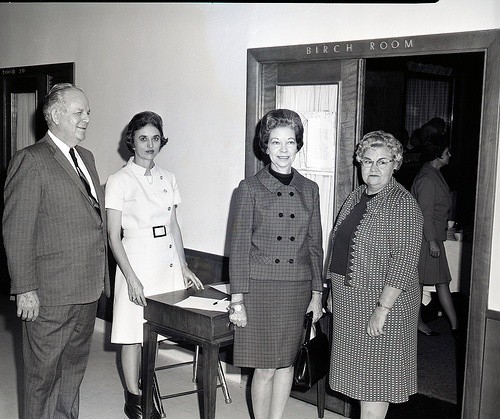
[[376, 301, 393, 309]]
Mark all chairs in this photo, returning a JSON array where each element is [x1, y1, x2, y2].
[[147, 282, 231, 419]]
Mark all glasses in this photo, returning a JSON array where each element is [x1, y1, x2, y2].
[[360, 157, 396, 168]]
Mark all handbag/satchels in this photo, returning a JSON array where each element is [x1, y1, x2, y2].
[[293, 311, 330, 393]]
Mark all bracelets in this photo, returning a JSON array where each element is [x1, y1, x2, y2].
[[226, 299, 244, 315], [311, 291, 323, 295]]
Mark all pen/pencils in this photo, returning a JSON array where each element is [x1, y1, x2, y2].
[[213, 295, 229, 305]]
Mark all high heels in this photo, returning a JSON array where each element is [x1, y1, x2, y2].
[[417, 328, 439, 336]]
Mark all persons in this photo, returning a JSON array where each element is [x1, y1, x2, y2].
[[3, 81, 110, 419], [102, 111, 205, 419], [408, 133, 470, 338], [325, 131, 423, 419], [224, 109, 324, 419]]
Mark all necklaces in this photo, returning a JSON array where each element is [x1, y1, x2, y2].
[[145, 173, 154, 184]]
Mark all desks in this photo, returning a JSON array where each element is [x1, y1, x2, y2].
[[143, 280, 331, 419]]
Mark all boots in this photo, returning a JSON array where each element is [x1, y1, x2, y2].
[[123, 391, 142, 419], [139, 385, 160, 419]]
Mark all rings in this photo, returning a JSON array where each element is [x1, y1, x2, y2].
[[132, 298, 137, 301]]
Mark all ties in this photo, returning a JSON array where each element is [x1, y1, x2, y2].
[[69, 147, 102, 221]]
